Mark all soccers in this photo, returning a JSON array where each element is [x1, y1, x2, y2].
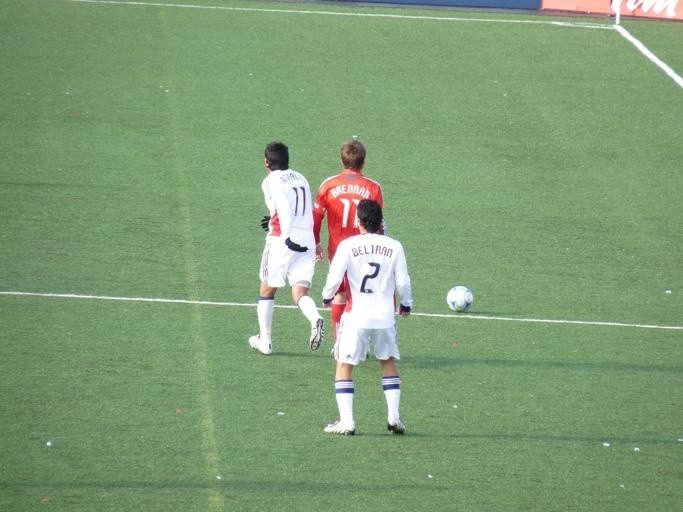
[[447, 286, 473, 311]]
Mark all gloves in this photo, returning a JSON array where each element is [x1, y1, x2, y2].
[[260, 216, 269, 232], [286, 237, 308, 252]]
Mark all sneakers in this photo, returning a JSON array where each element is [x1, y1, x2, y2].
[[324, 420, 356, 434], [310, 316, 325, 351], [249, 335, 273, 355], [388, 420, 405, 434]]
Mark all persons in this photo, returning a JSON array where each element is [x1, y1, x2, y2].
[[247, 141, 325, 357], [320, 198, 413, 436], [312, 138, 383, 355]]
